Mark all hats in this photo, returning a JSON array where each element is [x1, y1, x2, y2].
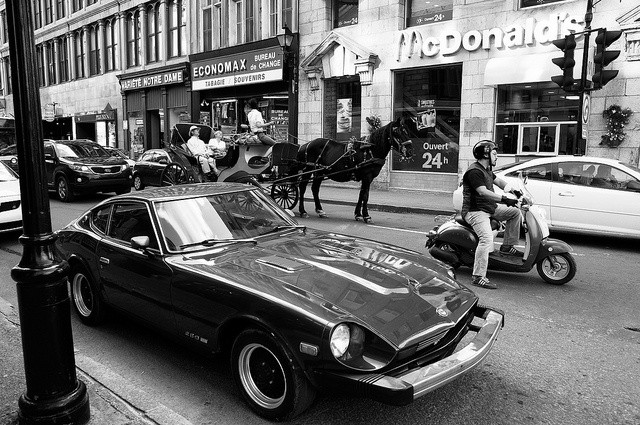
[[189, 126, 201, 136]]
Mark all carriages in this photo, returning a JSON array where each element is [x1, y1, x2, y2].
[[160, 115, 415, 224]]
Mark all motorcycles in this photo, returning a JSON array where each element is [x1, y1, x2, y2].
[[426, 174, 577, 287]]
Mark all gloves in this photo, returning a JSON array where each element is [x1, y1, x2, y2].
[[500, 195, 517, 207]]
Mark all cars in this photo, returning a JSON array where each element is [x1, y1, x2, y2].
[[41, 140, 133, 201], [131, 148, 188, 190], [0, 160, 23, 235], [54, 181, 504, 422], [451, 155, 640, 239]]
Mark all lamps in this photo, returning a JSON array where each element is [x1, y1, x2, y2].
[[276, 22, 295, 53], [201, 99, 209, 106]]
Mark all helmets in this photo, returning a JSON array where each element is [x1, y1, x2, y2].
[[473, 140, 498, 160]]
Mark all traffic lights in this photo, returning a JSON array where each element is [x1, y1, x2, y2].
[[551, 36, 576, 87], [593, 28, 622, 86]]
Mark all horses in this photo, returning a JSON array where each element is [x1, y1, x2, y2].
[[289, 116, 415, 225]]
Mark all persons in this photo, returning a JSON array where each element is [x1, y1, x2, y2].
[[461, 139, 525, 290], [591, 165, 618, 188], [209, 129, 228, 158], [186, 125, 218, 177], [246, 99, 278, 146]]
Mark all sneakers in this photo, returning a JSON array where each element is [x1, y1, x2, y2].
[[500, 245, 524, 257], [471, 276, 496, 289]]
